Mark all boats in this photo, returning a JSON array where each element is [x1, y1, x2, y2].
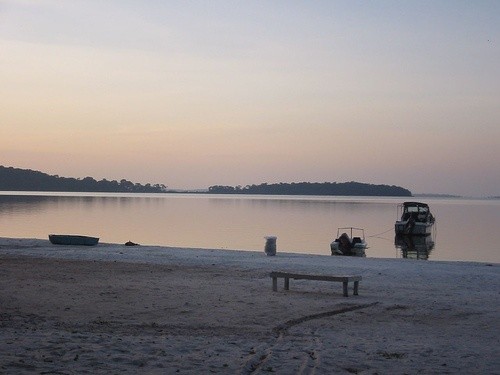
[[330, 227, 367, 256], [48, 235, 99, 246], [395, 202, 435, 236]]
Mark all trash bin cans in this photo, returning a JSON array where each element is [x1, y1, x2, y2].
[[264, 236, 279, 256]]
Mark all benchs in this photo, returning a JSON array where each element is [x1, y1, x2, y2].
[[269, 270, 363, 299]]
